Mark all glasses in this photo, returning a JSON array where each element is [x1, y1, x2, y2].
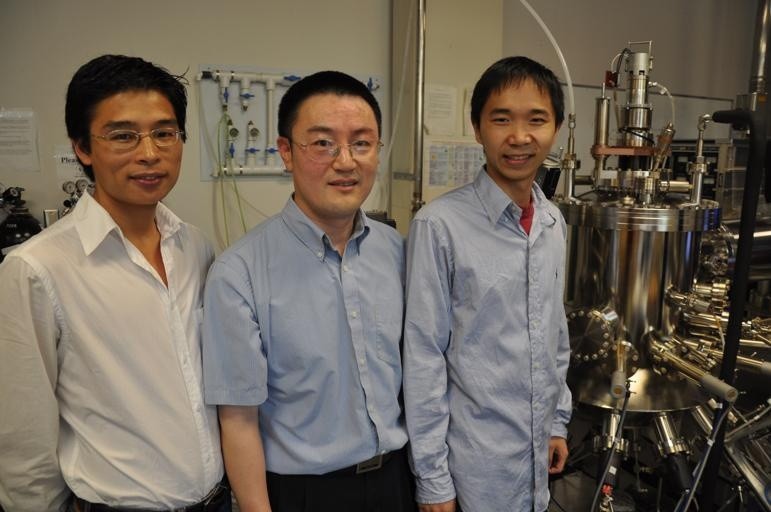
[[288, 133, 384, 163], [89, 126, 185, 149]]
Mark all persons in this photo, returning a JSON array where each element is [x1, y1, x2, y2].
[[0, 53, 235, 512], [402, 54, 574, 512], [194, 70, 461, 512]]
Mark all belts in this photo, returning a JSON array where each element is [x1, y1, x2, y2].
[[355, 453, 382, 474]]
